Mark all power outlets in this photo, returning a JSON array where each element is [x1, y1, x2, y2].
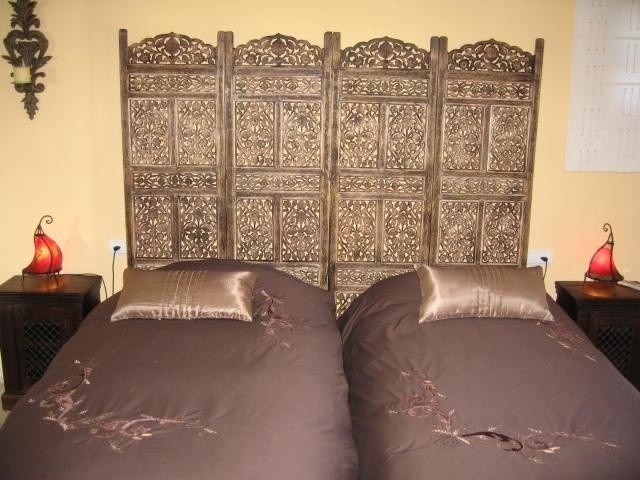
[[110, 241, 125, 255], [536, 251, 552, 264]]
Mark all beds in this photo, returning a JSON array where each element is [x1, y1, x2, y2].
[[1, 259, 360, 480], [337, 264, 640, 480]]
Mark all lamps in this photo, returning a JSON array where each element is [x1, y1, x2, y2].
[[22, 215, 62, 286], [583, 223, 624, 296]]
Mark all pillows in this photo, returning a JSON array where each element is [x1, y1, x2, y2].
[[110, 268, 258, 322], [413, 264, 555, 324]]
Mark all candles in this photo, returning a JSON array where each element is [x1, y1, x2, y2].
[[13, 58, 31, 83]]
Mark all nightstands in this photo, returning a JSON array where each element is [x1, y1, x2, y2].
[[555, 281, 640, 393], [1, 275, 102, 410]]
